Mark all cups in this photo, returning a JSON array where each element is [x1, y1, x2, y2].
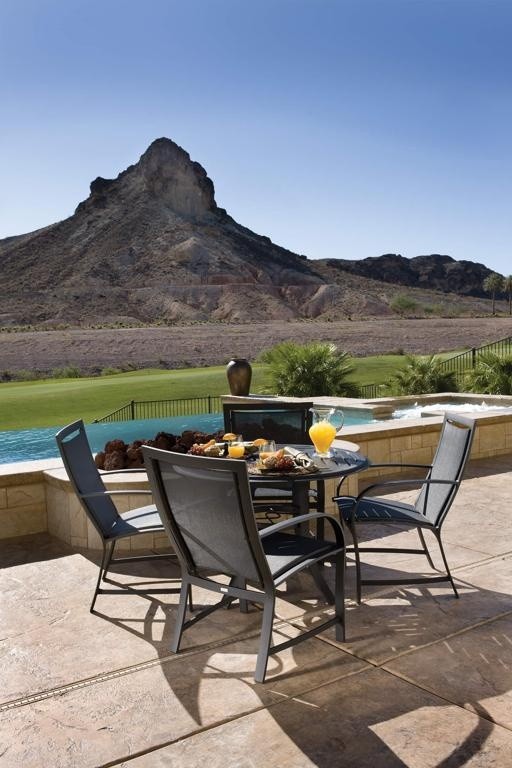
[[259, 439, 276, 461], [228, 434, 245, 458]]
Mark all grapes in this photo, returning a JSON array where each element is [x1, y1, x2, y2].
[[188, 445, 203, 455], [277, 457, 294, 468]]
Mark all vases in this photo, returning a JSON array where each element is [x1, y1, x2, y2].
[[225, 356, 253, 396]]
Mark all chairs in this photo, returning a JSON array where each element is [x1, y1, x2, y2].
[[55, 419, 195, 614], [221, 400, 325, 568], [334, 412, 478, 603], [138, 444, 347, 683]]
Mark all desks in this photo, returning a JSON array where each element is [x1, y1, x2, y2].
[[169, 444, 371, 609]]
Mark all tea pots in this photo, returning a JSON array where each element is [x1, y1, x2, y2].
[[307, 407, 345, 459]]
